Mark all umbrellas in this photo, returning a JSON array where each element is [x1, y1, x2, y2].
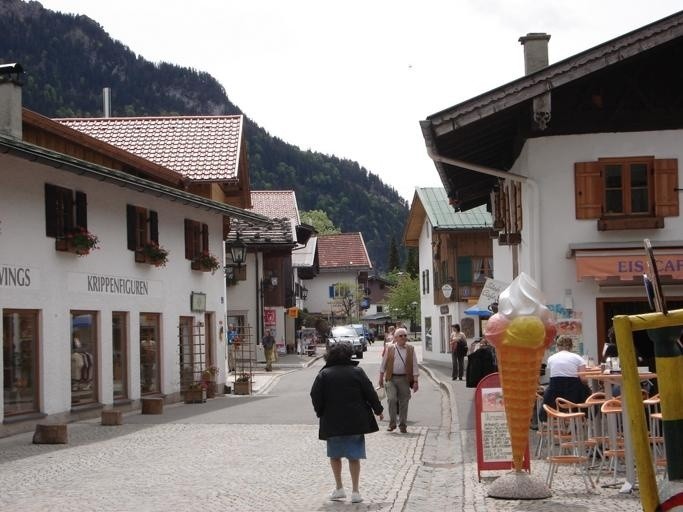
[[73, 315, 93, 327], [463, 303, 491, 317]]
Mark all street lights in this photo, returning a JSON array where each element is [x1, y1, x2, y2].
[[411, 301, 418, 339]]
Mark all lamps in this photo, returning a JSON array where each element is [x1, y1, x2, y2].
[[260, 271, 278, 294], [295, 285, 309, 300], [225, 231, 247, 273]]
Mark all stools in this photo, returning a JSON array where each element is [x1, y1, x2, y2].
[[535, 365, 668, 494]]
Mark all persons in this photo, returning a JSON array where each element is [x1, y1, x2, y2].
[[262, 329, 276, 372], [228, 323, 238, 372], [310, 340, 384, 503], [603, 327, 621, 398], [379, 327, 419, 433], [382, 326, 395, 357], [449, 324, 468, 381], [538, 334, 598, 422], [140, 330, 157, 392]]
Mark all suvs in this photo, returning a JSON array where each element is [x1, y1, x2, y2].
[[347, 323, 365, 350], [322, 325, 362, 360]]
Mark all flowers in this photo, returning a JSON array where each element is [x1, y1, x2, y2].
[[196, 252, 222, 275], [184, 383, 208, 390], [200, 363, 222, 375], [58, 228, 101, 257], [235, 370, 256, 382], [139, 240, 170, 268]]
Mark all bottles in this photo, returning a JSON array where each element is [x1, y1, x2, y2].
[[588, 358, 593, 367]]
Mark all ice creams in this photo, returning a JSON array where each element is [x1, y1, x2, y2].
[[483, 268, 559, 475]]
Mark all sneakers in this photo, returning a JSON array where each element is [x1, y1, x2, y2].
[[350, 491, 362, 503], [387, 425, 396, 431], [453, 377, 462, 380], [329, 488, 345, 498], [400, 426, 407, 433]]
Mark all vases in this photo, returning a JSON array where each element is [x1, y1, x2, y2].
[[202, 375, 216, 398], [135, 251, 151, 264], [191, 262, 211, 272], [234, 378, 252, 394], [184, 391, 207, 404], [56, 240, 73, 252]]
